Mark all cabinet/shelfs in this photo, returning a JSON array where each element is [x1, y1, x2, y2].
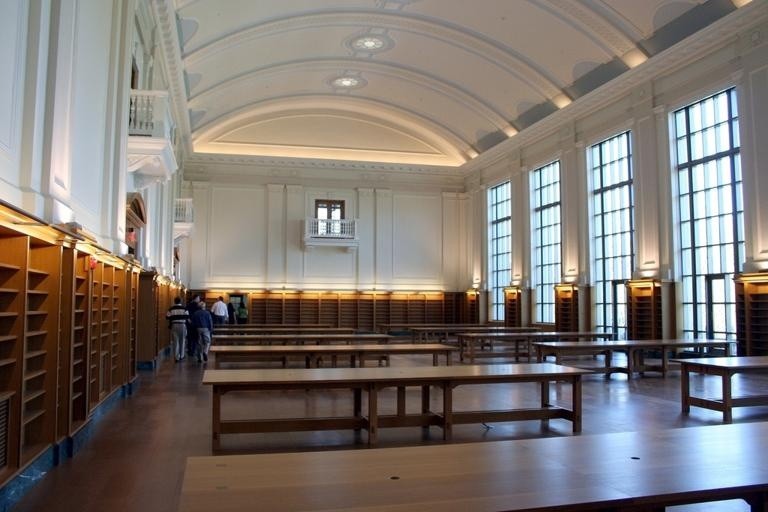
[[247, 291, 480, 335], [502, 286, 521, 327], [554, 282, 578, 342], [625, 280, 662, 340], [732, 273, 768, 356], [138, 269, 186, 370]]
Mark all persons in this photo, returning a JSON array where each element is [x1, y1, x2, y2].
[[227, 303, 236, 324], [238, 302, 248, 324], [193, 301, 213, 363], [185, 295, 200, 357], [210, 296, 229, 324], [166, 297, 191, 362]]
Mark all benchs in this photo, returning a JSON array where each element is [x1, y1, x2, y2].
[[377, 322, 618, 363], [201, 361, 595, 449], [668, 354, 768, 424], [209, 323, 459, 394], [178, 421, 767, 511], [531, 338, 739, 383]]
